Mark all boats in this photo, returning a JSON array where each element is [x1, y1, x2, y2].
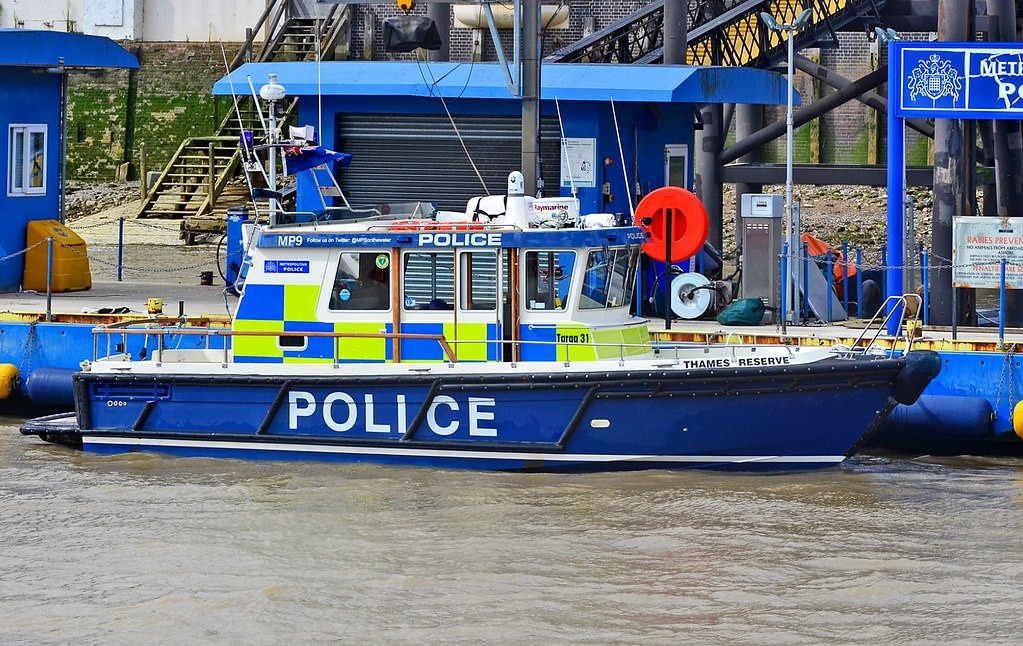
[[17, 71, 943, 473]]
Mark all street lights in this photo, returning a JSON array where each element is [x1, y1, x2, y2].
[[760, 8, 812, 324]]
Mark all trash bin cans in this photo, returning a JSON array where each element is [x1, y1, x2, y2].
[[24, 219, 92, 292]]
